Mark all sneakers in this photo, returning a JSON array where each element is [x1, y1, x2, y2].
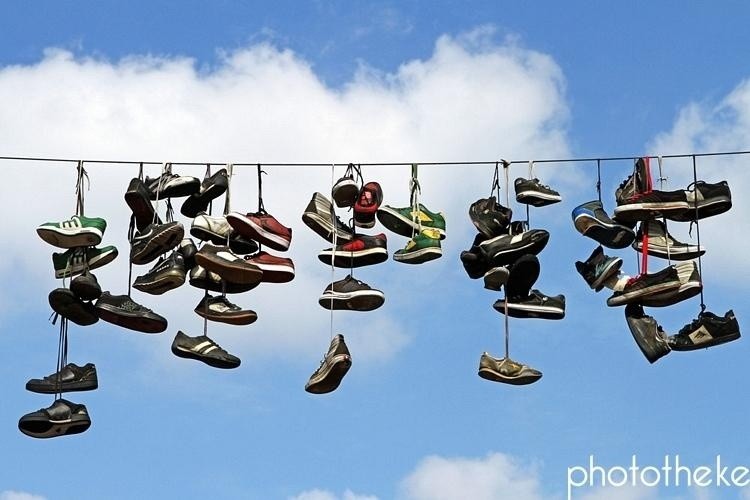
[[668, 310, 740, 351], [625, 305, 673, 364], [25, 362, 98, 394], [35, 156, 742, 333], [18, 397, 91, 438], [304, 334, 354, 393], [478, 351, 543, 386], [170, 331, 240, 369]]
